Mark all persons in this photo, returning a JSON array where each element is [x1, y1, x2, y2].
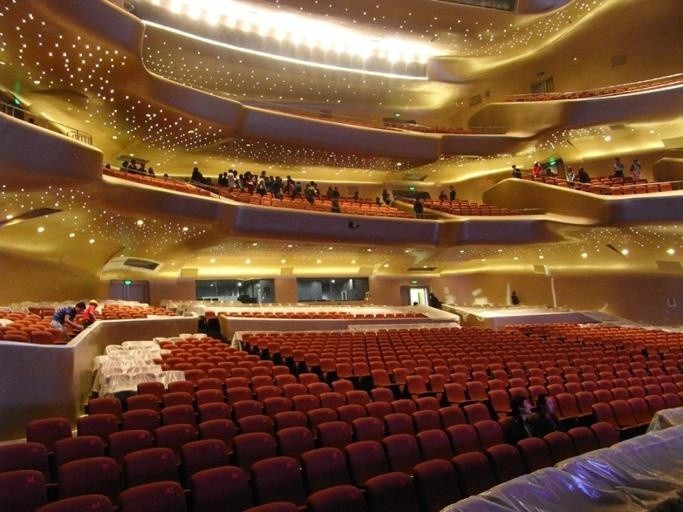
[[217, 169, 320, 205], [438, 185, 456, 208], [82, 299, 99, 327], [327, 186, 340, 198], [505, 395, 536, 447], [106, 158, 155, 178], [429, 293, 440, 311], [511, 290, 521, 305], [413, 196, 424, 218], [512, 156, 644, 189], [51, 300, 87, 335], [184, 167, 214, 190], [379, 189, 392, 205], [531, 392, 563, 436]]
